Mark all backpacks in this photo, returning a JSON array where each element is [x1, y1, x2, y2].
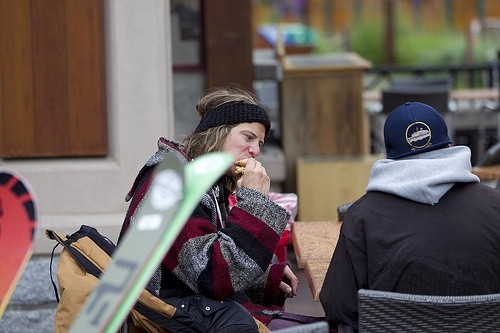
[[45, 223, 207, 333]]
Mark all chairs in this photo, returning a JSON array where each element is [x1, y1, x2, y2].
[[47, 227, 329, 333], [357, 289, 500, 332]]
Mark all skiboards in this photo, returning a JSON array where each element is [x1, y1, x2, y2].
[[66, 150, 237, 333], [0, 171, 38, 320]]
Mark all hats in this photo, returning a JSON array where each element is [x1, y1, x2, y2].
[[384, 101, 452, 158]]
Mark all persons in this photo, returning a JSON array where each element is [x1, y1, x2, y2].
[[116, 90, 299, 333], [318, 101, 500, 333]]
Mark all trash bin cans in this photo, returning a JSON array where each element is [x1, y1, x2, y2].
[[280, 51, 375, 191]]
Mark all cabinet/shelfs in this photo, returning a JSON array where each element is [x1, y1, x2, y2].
[[279, 55, 372, 194]]
